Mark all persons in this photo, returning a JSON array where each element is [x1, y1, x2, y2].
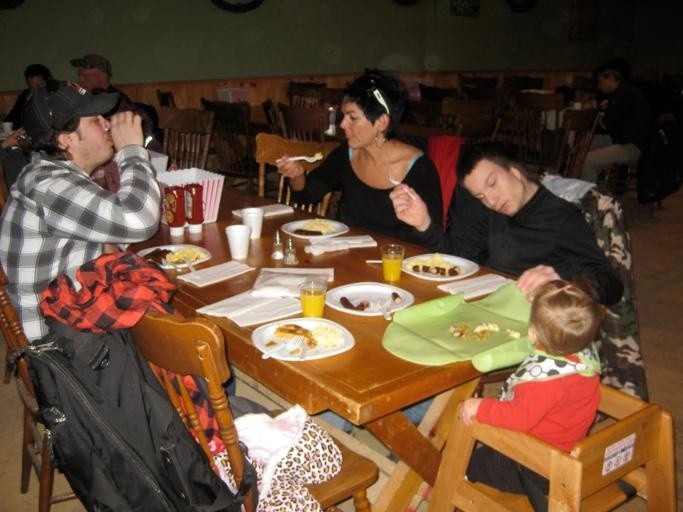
[[0, 55, 172, 192], [277, 74, 443, 248], [571, 57, 651, 182], [460, 280, 601, 496], [401, 136, 624, 426], [0, 81, 235, 403]]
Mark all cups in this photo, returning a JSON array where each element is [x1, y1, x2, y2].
[[1, 122, 13, 133], [225, 224, 251, 260], [240, 208, 264, 239], [297, 279, 326, 317], [573, 102, 582, 110], [103, 117, 153, 149], [379, 244, 405, 282]]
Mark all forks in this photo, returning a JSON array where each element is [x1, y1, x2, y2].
[[375, 299, 391, 322], [259, 334, 303, 360], [161, 258, 183, 272], [275, 155, 318, 164], [387, 172, 415, 200]]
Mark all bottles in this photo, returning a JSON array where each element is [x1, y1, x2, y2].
[[284, 236, 299, 266], [163, 183, 203, 237], [325, 106, 336, 135], [271, 229, 284, 260]]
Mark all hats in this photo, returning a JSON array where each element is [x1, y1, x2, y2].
[[21, 81, 120, 144], [69, 54, 112, 74]]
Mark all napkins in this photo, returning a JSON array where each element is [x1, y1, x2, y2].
[[435, 272, 508, 300], [228, 296, 303, 328], [195, 290, 282, 317], [176, 260, 255, 288], [251, 271, 308, 298], [260, 267, 334, 282], [307, 233, 379, 255]]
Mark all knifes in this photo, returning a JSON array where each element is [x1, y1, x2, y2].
[[181, 256, 199, 279]]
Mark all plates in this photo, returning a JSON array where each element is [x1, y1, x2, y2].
[[251, 317, 356, 362], [135, 243, 210, 269], [402, 253, 480, 282], [279, 218, 349, 240], [323, 281, 415, 317], [0, 133, 7, 140]]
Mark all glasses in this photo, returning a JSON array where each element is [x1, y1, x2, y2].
[[362, 79, 391, 114]]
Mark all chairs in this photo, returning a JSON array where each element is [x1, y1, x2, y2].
[[0, 65, 681, 232], [1, 277, 79, 512], [116, 308, 380, 511], [427, 375, 677, 512]]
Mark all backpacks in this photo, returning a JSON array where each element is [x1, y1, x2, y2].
[[4, 316, 261, 508]]
